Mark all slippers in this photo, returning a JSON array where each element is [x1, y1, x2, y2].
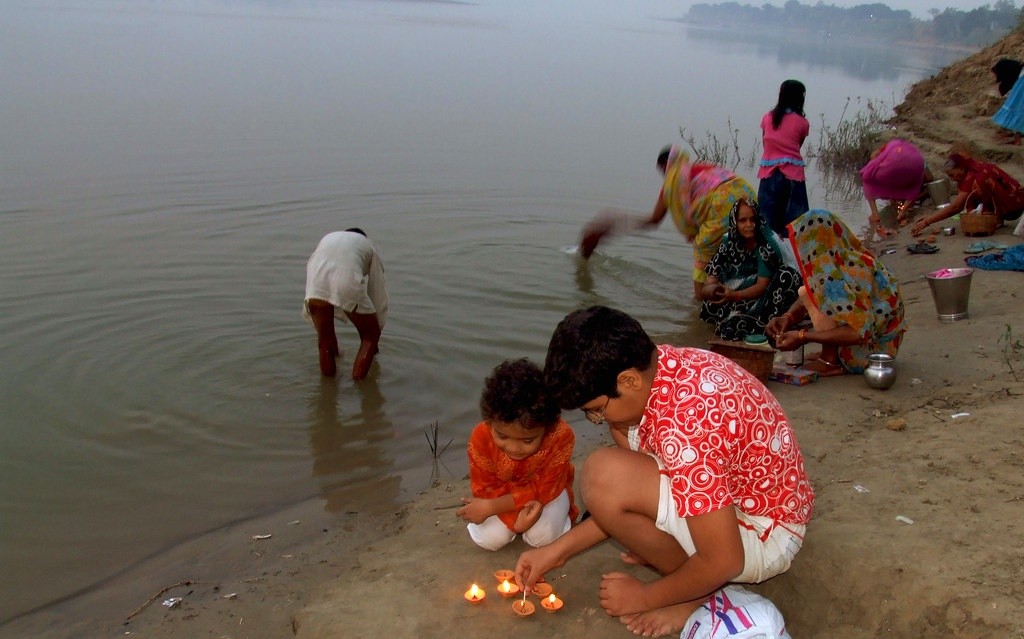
[[907, 243, 940, 252], [963, 241, 1008, 254]]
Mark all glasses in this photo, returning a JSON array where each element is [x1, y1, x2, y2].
[[585, 396, 611, 425]]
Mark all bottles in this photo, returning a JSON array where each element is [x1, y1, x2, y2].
[[745, 335, 772, 350], [785, 325, 804, 367]]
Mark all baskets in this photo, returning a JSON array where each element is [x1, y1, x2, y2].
[[960, 188, 998, 237]]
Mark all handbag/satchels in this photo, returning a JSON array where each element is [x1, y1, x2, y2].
[[680, 585, 792, 639]]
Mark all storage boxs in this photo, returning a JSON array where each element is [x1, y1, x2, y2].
[[768, 363, 818, 387]]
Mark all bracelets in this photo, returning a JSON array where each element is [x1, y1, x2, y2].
[[784, 312, 797, 324], [924, 219, 929, 226], [799, 329, 807, 342]]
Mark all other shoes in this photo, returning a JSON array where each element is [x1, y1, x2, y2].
[[801, 358, 844, 376]]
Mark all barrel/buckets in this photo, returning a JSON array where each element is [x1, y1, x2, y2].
[[925, 178, 950, 209], [924, 268, 974, 320]]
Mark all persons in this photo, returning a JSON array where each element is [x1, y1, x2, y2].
[[990, 66, 1024, 146], [859, 139, 933, 226], [766, 206, 907, 376], [701, 197, 802, 340], [757, 80, 809, 238], [456, 357, 580, 552], [631, 143, 757, 302], [514, 304, 815, 638], [910, 151, 1024, 235], [301, 227, 389, 378]]
